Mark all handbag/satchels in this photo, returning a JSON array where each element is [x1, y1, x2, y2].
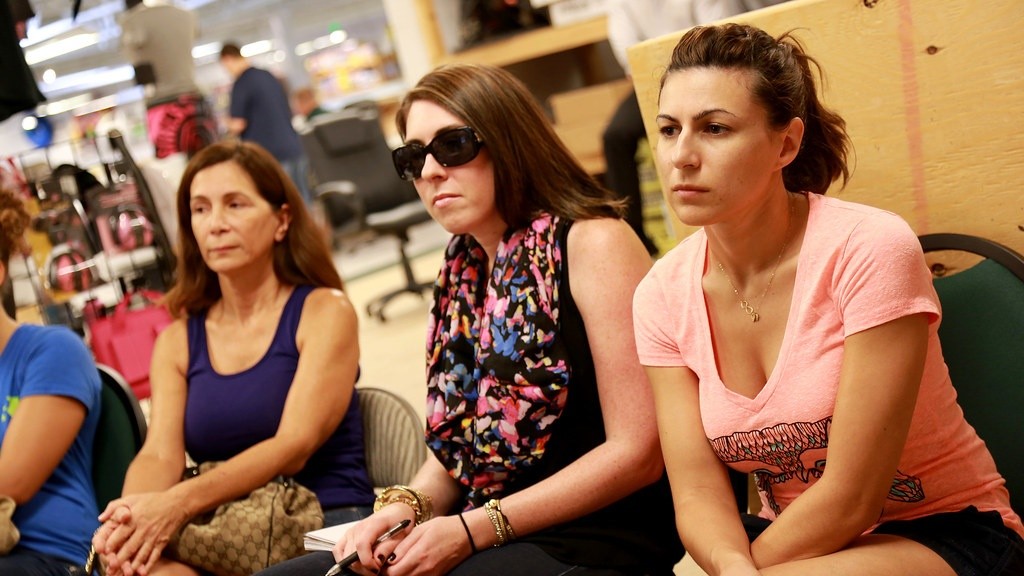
[[164, 466, 325, 576]]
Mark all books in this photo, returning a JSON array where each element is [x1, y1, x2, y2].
[[303, 520, 402, 551]]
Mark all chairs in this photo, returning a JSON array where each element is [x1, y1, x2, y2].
[[354, 388, 425, 489], [89, 365, 147, 515], [300, 100, 435, 322], [917, 232, 1024, 525]]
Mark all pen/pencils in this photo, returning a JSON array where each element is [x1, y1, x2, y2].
[[323, 519, 412, 576]]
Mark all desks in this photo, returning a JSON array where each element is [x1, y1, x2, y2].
[[452, 16, 607, 72]]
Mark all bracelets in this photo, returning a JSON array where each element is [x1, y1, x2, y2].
[[459, 513, 477, 554], [373, 484, 435, 526], [483, 498, 515, 547]]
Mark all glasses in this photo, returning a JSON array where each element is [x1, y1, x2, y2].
[[391, 125, 483, 182]]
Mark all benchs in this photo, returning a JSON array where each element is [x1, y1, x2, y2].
[[549, 78, 633, 177]]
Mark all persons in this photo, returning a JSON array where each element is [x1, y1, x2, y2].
[[632, 21, 1024, 576], [0, 187, 104, 576], [219, 42, 334, 248], [599, 0, 779, 254], [91, 140, 378, 576], [256, 64, 749, 576]]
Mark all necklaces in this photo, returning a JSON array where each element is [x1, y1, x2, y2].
[[713, 197, 794, 323]]
